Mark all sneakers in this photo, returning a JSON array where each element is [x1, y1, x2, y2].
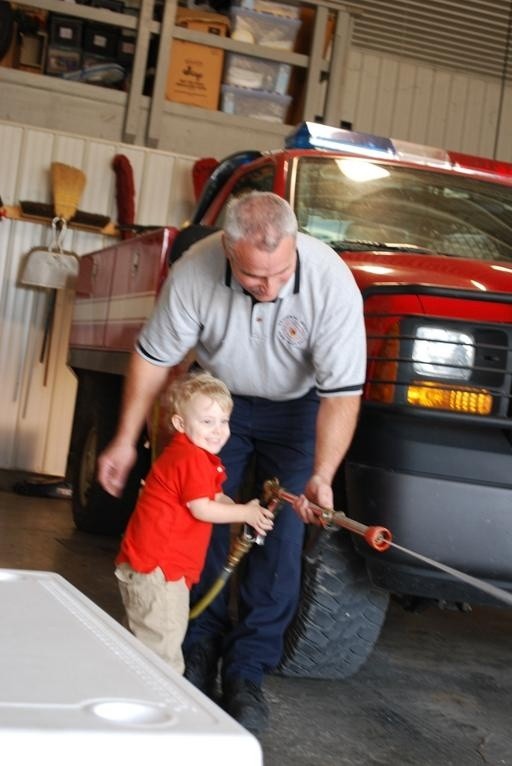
[[183, 639, 218, 692], [222, 676, 270, 731]]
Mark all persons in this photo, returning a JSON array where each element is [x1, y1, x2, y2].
[[114, 373, 276, 676], [95, 190, 367, 734]]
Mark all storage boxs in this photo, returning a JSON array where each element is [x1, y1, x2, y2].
[[165, 2, 303, 123]]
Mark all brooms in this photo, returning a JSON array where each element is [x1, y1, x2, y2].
[[40, 160, 86, 387]]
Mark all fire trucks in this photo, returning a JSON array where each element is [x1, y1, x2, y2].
[[60, 120, 512, 681]]
